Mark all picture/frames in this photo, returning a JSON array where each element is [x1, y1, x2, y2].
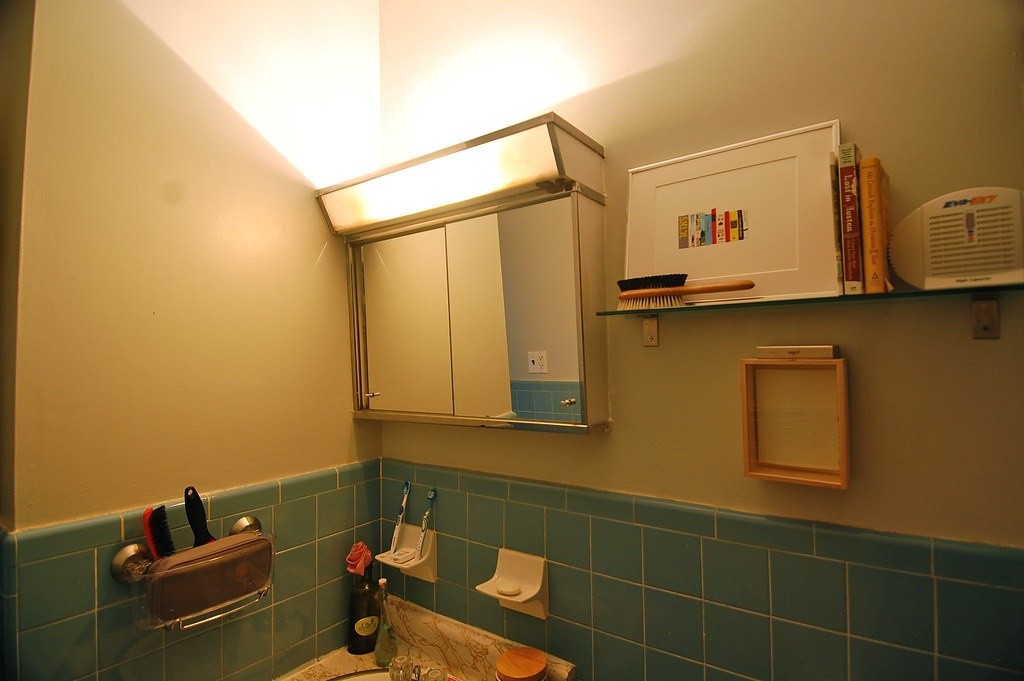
[[625, 119, 843, 306]]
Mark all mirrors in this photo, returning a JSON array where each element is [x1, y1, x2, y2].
[[353, 197, 583, 424]]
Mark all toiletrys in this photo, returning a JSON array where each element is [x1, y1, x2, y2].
[[374, 577, 398, 667]]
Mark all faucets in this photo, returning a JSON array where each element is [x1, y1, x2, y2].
[[410, 664, 422, 681]]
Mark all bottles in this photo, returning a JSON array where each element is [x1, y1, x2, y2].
[[376, 579, 396, 667], [347, 539, 377, 654]]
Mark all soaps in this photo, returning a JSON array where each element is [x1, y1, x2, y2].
[[497, 580, 523, 596]]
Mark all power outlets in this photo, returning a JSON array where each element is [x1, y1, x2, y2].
[[527, 350, 547, 374]]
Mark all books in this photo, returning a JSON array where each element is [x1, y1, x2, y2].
[[677, 208, 747, 249], [828, 143, 894, 296]]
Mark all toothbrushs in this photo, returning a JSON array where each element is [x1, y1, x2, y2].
[[391, 480, 411, 555], [415, 485, 436, 562]]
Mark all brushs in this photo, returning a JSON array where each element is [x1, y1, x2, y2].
[[616, 274, 755, 310], [184, 486, 217, 548], [143, 505, 175, 561]]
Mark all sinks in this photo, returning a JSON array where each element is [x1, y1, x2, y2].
[[325, 668, 391, 681]]
